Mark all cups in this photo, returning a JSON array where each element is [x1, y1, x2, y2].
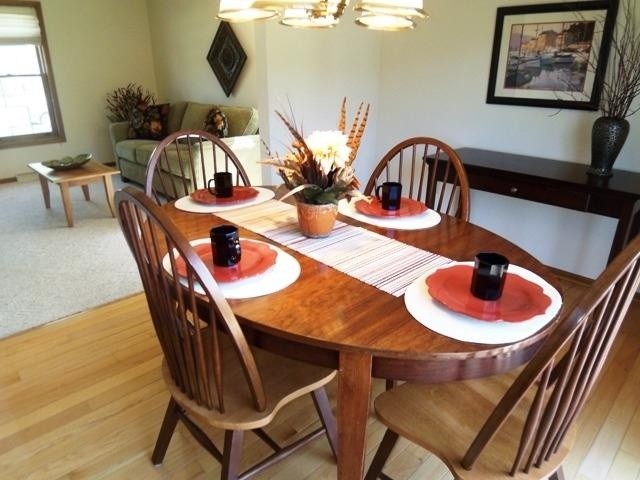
[[209, 225, 242, 267], [209, 172, 234, 199], [376, 182, 403, 210], [470, 253, 509, 300]]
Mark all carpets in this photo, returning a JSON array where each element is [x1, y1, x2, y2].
[[1, 163, 170, 341]]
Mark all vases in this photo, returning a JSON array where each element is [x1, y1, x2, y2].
[[586, 116, 629, 178]]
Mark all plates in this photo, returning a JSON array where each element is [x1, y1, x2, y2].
[[41, 151, 93, 171], [172, 240, 278, 284], [355, 197, 428, 217], [425, 267, 552, 321], [191, 186, 259, 206]]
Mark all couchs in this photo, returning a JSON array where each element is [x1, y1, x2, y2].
[[109, 100, 262, 201]]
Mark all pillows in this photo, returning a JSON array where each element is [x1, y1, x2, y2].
[[202, 108, 229, 143], [128, 102, 170, 141]]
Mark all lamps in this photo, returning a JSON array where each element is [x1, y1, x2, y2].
[[217, 0, 430, 33]]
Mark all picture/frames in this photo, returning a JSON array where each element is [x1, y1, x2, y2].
[[485, 0, 620, 112]]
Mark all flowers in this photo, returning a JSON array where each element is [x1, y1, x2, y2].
[[263, 99, 371, 202]]
